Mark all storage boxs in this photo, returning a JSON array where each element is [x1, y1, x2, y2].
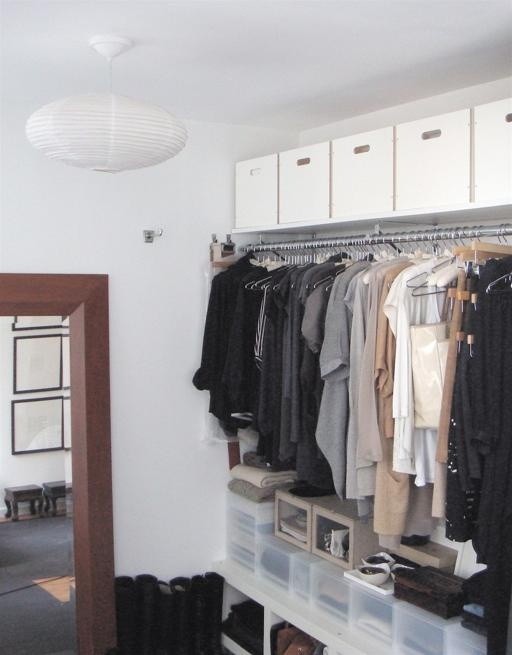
[[313, 563, 353, 621], [390, 598, 460, 655], [309, 497, 388, 569], [341, 571, 394, 655], [226, 487, 280, 574], [236, 96, 512, 229], [446, 621, 487, 655], [254, 532, 311, 603], [272, 487, 336, 554]]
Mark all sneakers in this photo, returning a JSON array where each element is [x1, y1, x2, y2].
[[277, 626, 317, 655]]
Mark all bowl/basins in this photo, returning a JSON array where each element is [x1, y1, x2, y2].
[[357, 551, 416, 585]]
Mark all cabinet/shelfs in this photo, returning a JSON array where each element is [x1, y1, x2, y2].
[[225, 567, 363, 655], [214, 562, 360, 655]]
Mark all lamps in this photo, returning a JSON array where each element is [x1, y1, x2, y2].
[[24, 35, 187, 173]]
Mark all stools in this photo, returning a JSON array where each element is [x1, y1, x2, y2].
[[43, 481, 65, 517], [4, 484, 45, 521]]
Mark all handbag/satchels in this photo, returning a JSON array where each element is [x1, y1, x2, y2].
[[410, 322, 449, 430]]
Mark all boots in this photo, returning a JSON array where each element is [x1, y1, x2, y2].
[[111, 572, 225, 655]]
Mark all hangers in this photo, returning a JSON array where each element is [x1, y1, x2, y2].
[[242, 223, 512, 296]]
